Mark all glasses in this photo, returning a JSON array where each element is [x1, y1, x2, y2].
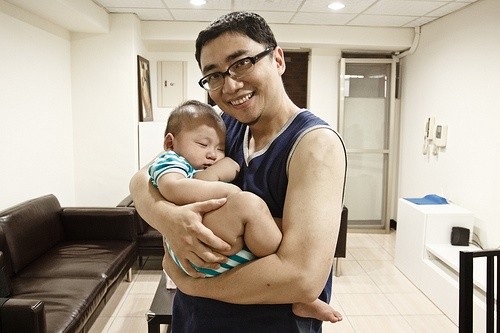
[[198, 47, 273, 91]]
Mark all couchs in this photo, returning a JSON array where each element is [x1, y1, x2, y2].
[[0, 193, 164, 333]]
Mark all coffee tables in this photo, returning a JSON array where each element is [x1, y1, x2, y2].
[[147, 271, 180, 333]]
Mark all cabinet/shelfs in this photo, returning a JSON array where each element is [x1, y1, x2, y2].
[[394, 197, 500, 333]]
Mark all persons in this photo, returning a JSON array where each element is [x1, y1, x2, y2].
[[147, 99, 343, 323], [129, 10, 348, 333]]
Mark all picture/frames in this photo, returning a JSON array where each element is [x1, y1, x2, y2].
[[137, 54, 153, 121]]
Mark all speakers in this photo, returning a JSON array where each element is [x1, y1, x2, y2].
[[451, 227, 469, 246]]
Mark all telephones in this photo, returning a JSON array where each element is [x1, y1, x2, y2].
[[434, 125, 447, 146], [424, 115, 434, 140]]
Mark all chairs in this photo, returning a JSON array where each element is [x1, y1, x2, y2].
[[458, 249, 500, 333]]
